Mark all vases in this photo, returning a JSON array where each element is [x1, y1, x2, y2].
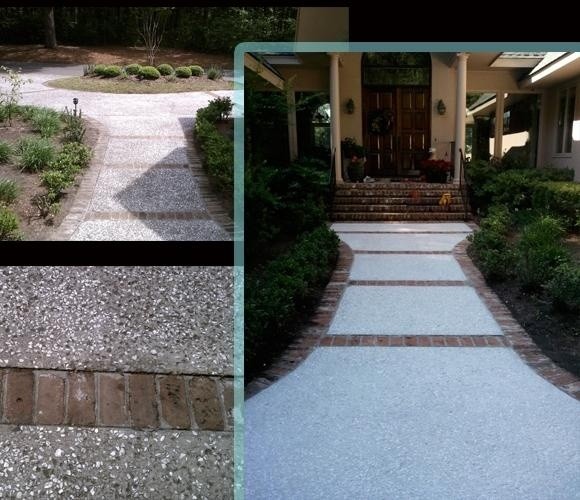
[[425, 172, 448, 183], [347, 167, 364, 182]]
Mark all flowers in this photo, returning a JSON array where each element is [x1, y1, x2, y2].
[[346, 155, 368, 168], [420, 159, 451, 173]]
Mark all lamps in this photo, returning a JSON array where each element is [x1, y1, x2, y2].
[[437, 98, 446, 116], [345, 98, 355, 114]]
